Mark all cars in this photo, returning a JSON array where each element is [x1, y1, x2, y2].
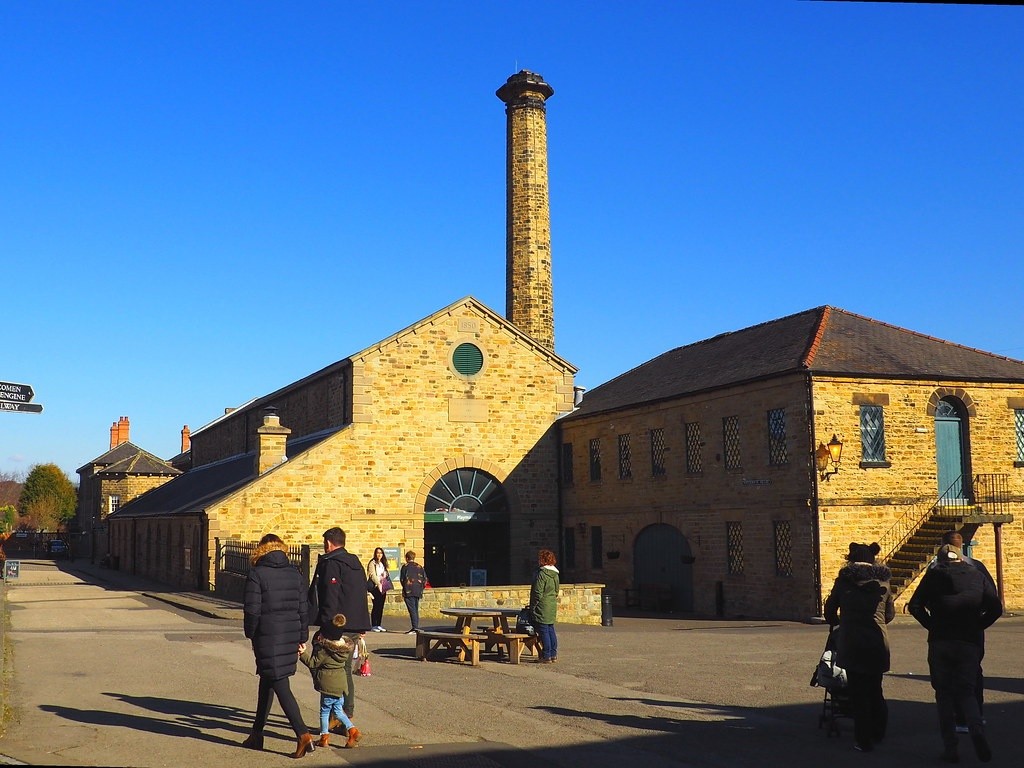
[[50, 538, 69, 555]]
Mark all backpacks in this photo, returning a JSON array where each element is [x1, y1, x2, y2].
[[516, 607, 536, 634]]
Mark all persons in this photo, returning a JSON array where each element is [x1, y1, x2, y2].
[[924, 530, 1003, 736], [297, 614, 364, 750], [367, 547, 391, 633], [303, 526, 372, 731], [906, 544, 1005, 764], [400, 551, 427, 635], [237, 533, 316, 761], [529, 548, 560, 664], [823, 541, 899, 753]]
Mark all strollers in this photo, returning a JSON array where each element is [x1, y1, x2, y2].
[[815, 620, 855, 737]]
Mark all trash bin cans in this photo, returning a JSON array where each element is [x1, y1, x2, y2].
[[602, 595, 613, 626], [5, 560, 20, 579], [111, 556, 120, 570], [470, 569, 486, 586]]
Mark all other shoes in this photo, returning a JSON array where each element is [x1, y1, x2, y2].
[[849, 743, 871, 752], [972, 729, 991, 762], [933, 749, 959, 763]]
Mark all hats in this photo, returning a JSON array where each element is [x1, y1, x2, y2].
[[320, 613, 346, 641], [937, 544, 961, 562], [847, 541, 880, 566]]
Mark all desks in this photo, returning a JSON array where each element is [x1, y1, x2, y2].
[[440, 608, 520, 662]]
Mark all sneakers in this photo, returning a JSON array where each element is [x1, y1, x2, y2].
[[534, 657, 552, 663], [404, 628, 417, 634], [552, 657, 558, 662], [372, 627, 381, 633], [379, 626, 386, 632]]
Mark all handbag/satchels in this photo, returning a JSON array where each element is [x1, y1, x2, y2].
[[350, 633, 370, 676], [381, 577, 393, 594], [366, 578, 376, 594]]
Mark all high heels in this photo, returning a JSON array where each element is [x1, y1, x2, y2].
[[290, 731, 316, 758], [241, 734, 264, 751]]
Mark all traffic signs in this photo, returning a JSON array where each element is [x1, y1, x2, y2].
[[0, 381, 45, 415]]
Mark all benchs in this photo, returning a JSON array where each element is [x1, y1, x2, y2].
[[414, 625, 463, 656], [420, 630, 488, 666], [503, 630, 546, 666], [475, 624, 521, 656]]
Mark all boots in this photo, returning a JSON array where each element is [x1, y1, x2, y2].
[[313, 733, 330, 746], [345, 728, 362, 748]]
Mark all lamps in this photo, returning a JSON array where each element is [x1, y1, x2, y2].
[[825, 434, 843, 483]]
